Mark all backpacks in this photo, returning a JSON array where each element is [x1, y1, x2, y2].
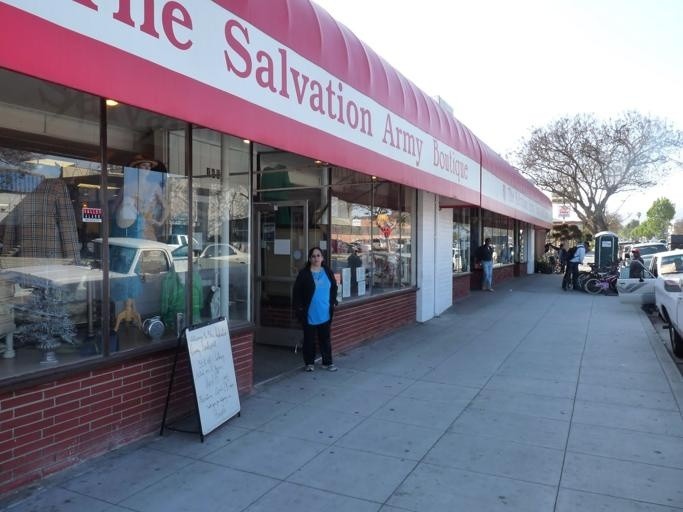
[[564, 245, 584, 261]]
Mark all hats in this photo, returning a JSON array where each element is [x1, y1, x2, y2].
[[128, 152, 158, 170], [630, 248, 640, 254], [583, 241, 590, 247]]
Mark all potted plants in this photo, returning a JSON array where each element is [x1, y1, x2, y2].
[[536, 255, 557, 275]]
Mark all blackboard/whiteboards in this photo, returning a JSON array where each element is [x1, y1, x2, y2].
[[185, 316, 241, 437]]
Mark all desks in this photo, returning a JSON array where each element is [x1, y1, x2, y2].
[[0, 267, 127, 360]]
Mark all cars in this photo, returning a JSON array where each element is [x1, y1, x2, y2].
[[14, 234, 248, 341], [618, 241, 683, 356], [331, 236, 514, 284]]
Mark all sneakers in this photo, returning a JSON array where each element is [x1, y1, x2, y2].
[[303, 364, 315, 372], [320, 363, 338, 372]]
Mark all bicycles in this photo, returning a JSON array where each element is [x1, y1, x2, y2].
[[577, 257, 626, 294]]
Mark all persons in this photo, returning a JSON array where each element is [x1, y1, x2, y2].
[[627, 247, 646, 279], [560, 240, 591, 291], [289, 246, 340, 373], [476, 237, 495, 293], [345, 249, 363, 285], [96, 160, 170, 303], [0, 173, 82, 294], [547, 242, 566, 274]]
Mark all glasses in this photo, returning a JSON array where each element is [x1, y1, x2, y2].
[[310, 254, 321, 258]]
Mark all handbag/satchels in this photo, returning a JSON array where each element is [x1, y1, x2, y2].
[[115, 194, 138, 229]]
